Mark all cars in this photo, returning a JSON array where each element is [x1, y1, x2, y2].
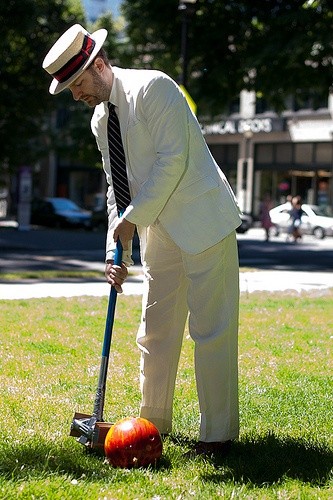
[[266, 203, 333, 240], [30, 197, 92, 230], [236, 215, 252, 234]]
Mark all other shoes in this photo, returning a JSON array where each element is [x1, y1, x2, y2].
[[182, 441, 226, 465]]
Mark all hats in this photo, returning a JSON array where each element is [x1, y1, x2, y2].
[[42, 24, 107, 95]]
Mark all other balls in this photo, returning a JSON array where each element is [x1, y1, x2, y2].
[[105, 416, 163, 468]]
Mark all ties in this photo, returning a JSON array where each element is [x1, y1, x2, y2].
[[107, 102, 131, 219]]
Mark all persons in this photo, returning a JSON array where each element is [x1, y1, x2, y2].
[[43, 24, 243, 462], [260, 177, 331, 243]]
